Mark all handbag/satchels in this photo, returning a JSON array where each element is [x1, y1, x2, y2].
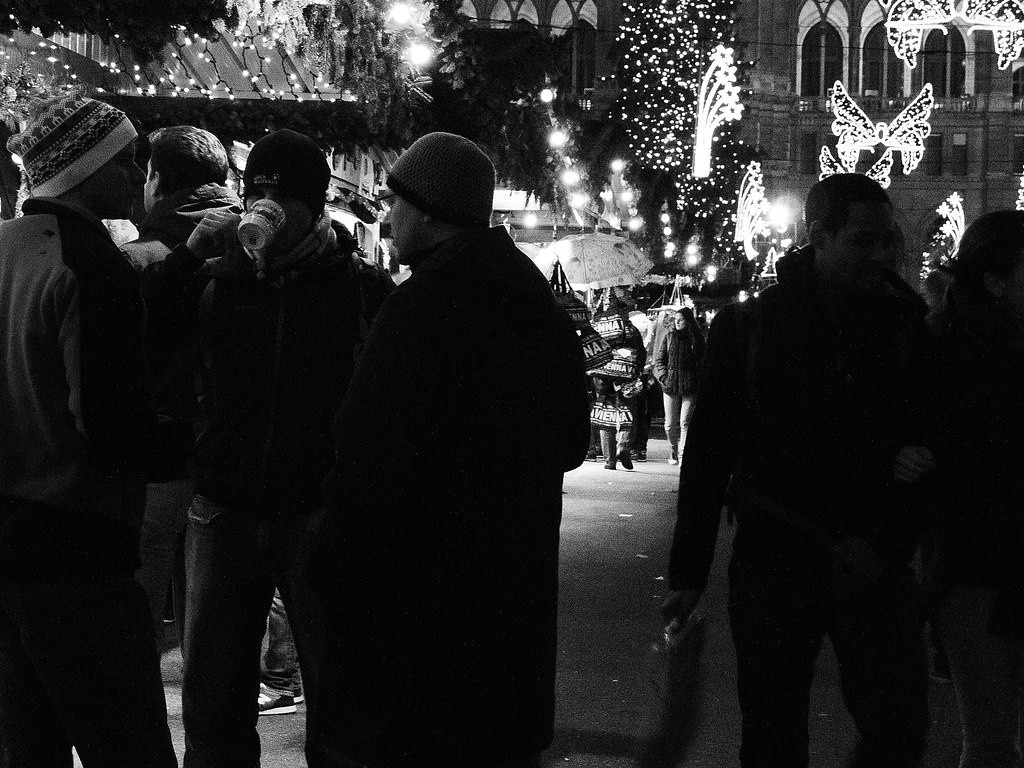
[[549, 261, 643, 430], [642, 613, 707, 763]]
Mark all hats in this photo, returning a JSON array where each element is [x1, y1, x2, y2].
[[6, 83, 139, 198], [242, 127, 331, 230], [386, 132, 495, 230]]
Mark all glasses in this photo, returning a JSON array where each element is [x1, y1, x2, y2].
[[376, 192, 399, 214]]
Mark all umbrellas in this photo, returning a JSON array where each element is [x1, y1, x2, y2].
[[535, 233, 654, 291]]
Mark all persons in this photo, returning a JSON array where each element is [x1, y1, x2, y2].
[[0, 95, 1024, 768]]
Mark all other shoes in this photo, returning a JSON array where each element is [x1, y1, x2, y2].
[[604, 461, 616, 469], [584, 455, 598, 462], [596, 452, 604, 458], [672, 452, 678, 464], [257, 682, 304, 715], [618, 451, 633, 470]]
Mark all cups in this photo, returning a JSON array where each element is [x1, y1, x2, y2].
[[237, 199, 287, 251]]
